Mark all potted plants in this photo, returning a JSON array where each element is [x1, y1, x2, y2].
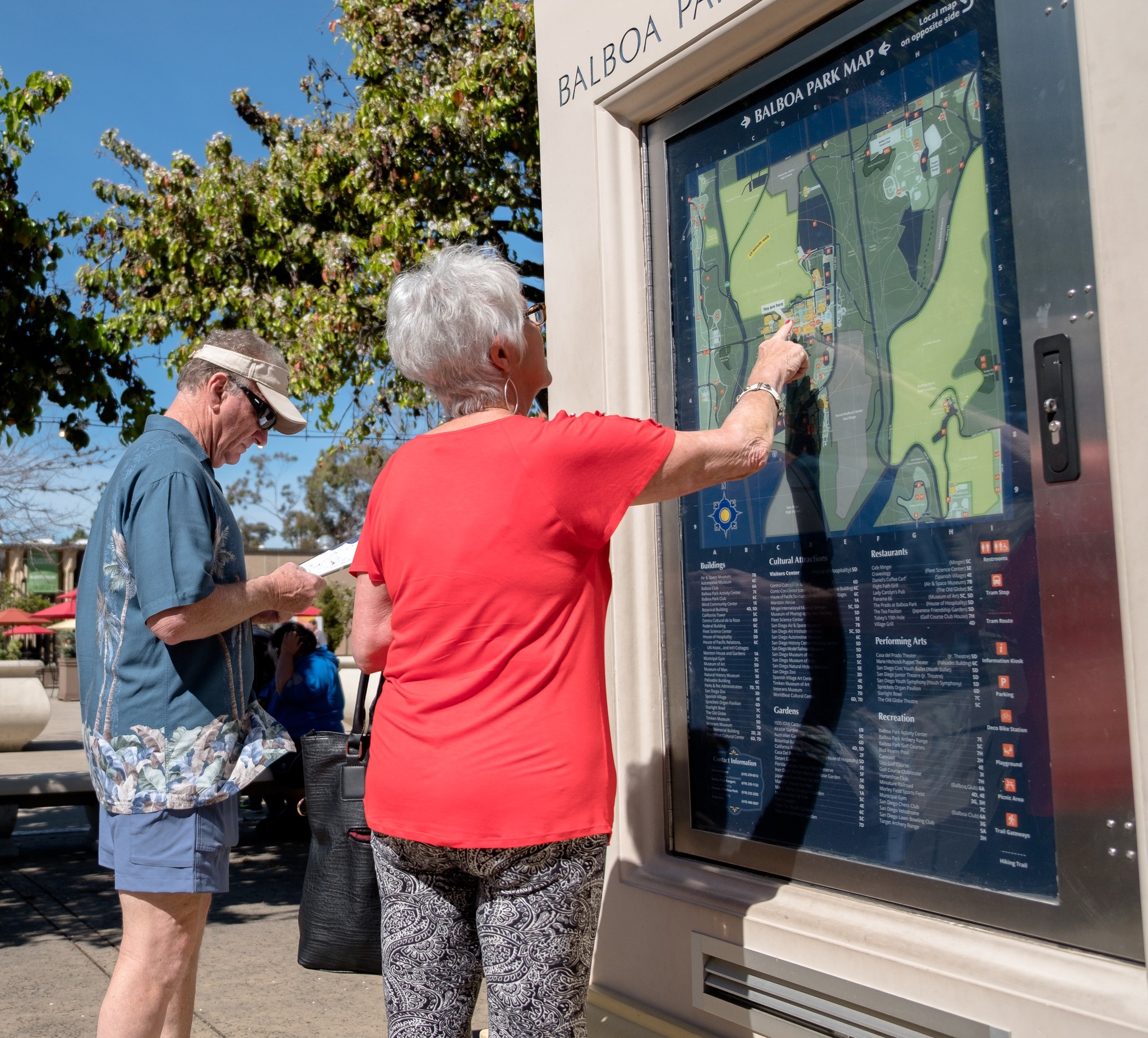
[[0, 638, 51, 752], [56, 628, 80, 702]]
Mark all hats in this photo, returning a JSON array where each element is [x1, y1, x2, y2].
[[189, 344, 307, 435]]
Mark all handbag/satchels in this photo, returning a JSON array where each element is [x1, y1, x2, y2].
[[296, 669, 386, 977]]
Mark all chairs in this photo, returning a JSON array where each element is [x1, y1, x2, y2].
[[48, 660, 59, 699]]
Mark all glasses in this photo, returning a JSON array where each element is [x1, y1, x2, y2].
[[522, 301, 547, 327], [225, 373, 278, 430]]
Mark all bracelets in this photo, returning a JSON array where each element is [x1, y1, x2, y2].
[[735, 382, 786, 422]]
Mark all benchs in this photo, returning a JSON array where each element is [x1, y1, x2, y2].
[[0, 771, 99, 839]]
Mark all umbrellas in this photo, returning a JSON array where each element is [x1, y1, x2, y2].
[[0, 587, 79, 636]]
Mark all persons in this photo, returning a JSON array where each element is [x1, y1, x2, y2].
[[79, 327, 346, 1038], [359, 245, 812, 1038]]
